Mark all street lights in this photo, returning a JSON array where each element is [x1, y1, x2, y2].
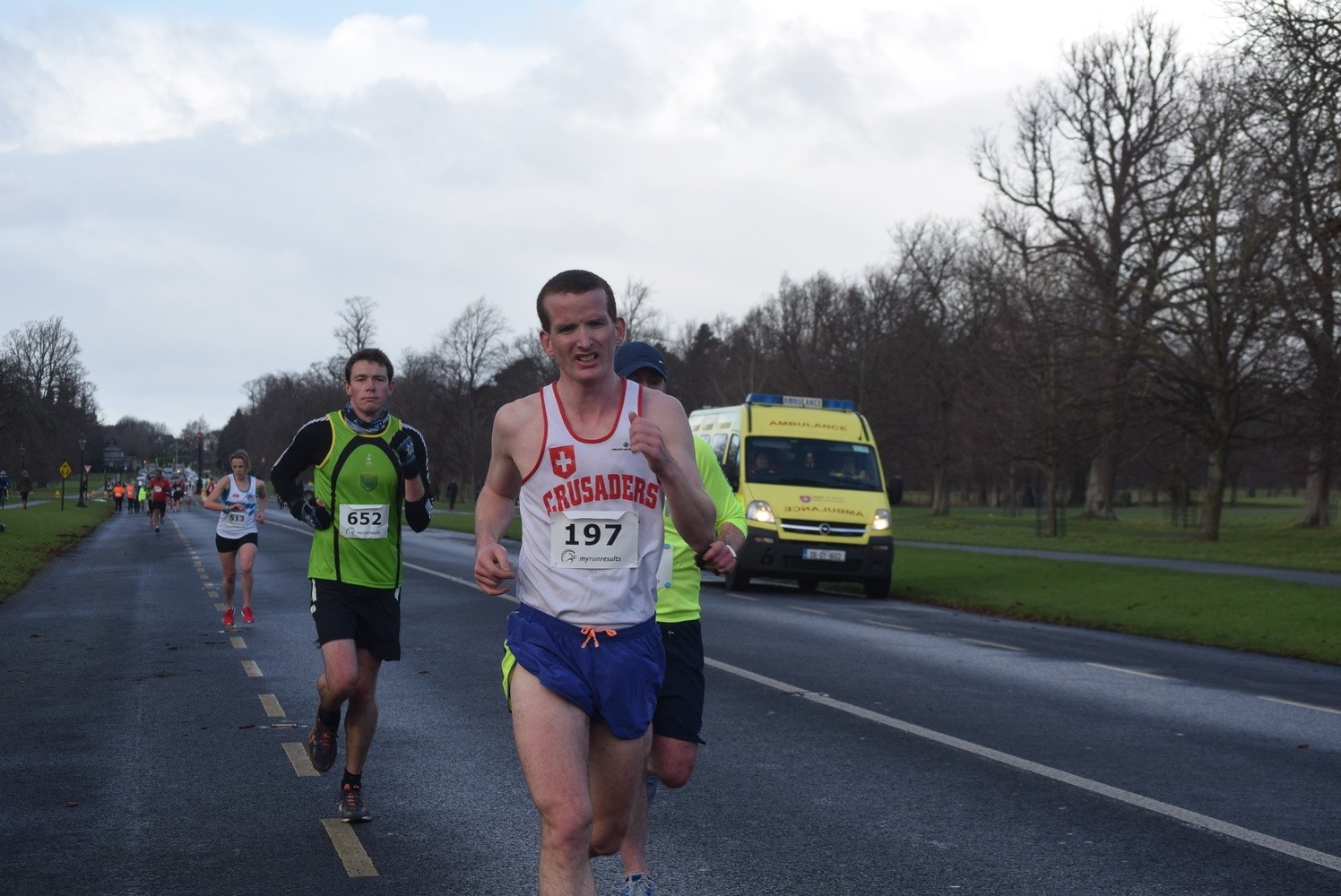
[[17, 444, 25, 471], [196, 427, 203, 477], [77, 433, 87, 508], [103, 457, 110, 491]]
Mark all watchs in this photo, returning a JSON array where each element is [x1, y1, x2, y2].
[[716, 539, 739, 560]]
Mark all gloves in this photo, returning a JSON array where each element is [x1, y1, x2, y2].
[[389, 429, 420, 479]]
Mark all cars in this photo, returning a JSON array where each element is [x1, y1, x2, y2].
[[136, 462, 199, 495]]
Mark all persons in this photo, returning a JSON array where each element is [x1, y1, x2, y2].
[[270, 348, 433, 824], [105, 463, 216, 533], [18, 469, 33, 509], [746, 447, 871, 488], [475, 269, 717, 896], [279, 477, 313, 510], [204, 449, 268, 627], [614, 342, 748, 896], [432, 475, 458, 513], [0, 470, 12, 500]]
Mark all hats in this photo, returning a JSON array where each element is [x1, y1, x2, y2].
[[614, 342, 667, 381]]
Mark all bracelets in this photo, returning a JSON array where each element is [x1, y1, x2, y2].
[[225, 504, 229, 514]]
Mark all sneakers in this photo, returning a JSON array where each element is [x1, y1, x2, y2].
[[339, 783, 372, 823], [308, 704, 341, 772], [222, 607, 235, 626], [241, 605, 255, 623], [622, 872, 656, 896], [647, 774, 658, 806]]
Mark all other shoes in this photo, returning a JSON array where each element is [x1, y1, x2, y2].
[[155, 525, 159, 533], [160, 517, 164, 524]]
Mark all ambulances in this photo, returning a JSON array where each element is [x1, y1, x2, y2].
[[688, 393, 895, 599]]
